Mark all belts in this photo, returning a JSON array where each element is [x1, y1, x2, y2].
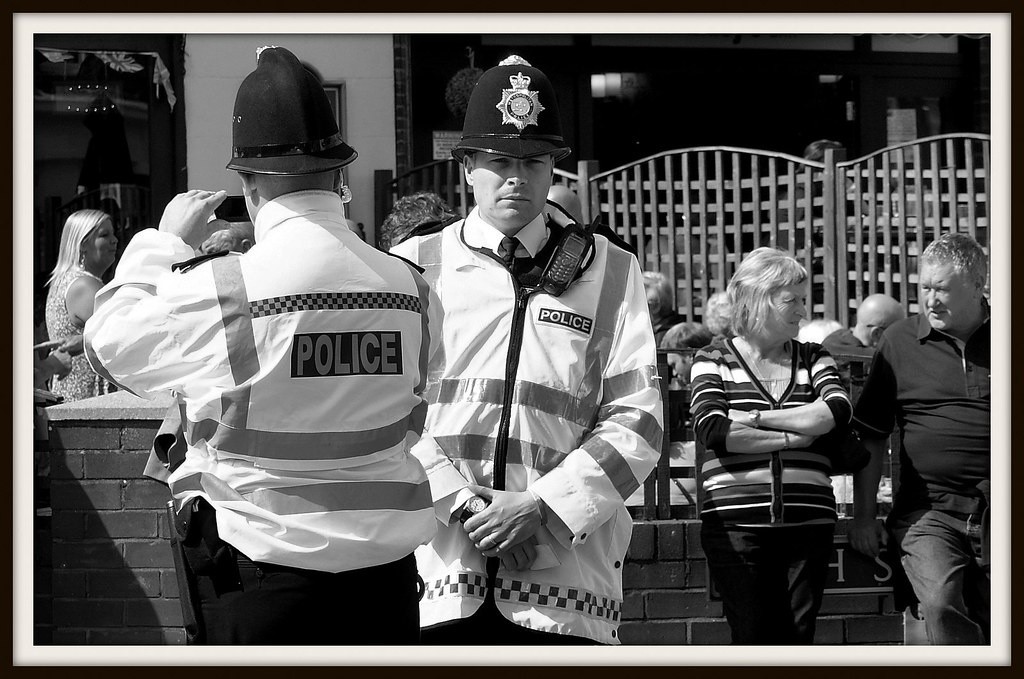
[[937, 509, 982, 525]]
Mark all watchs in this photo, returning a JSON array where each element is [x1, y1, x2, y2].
[[459, 496, 489, 524], [750, 409, 760, 427]]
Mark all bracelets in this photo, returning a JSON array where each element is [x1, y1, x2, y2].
[[529, 489, 548, 524], [783, 433, 789, 450]]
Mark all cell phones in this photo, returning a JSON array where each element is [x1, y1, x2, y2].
[[214, 196, 252, 223]]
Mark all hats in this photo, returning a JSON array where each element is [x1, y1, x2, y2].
[[451, 55, 571, 164], [226, 44, 358, 176]]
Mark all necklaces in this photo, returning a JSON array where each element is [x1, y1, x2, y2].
[[747, 350, 782, 392]]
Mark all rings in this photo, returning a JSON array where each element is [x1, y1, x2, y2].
[[497, 546, 502, 551], [488, 535, 497, 544]]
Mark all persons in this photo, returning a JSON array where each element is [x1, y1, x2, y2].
[[34, 209, 119, 402], [380, 193, 457, 251], [548, 186, 904, 439], [687, 247, 854, 646], [851, 234, 990, 645], [85, 47, 444, 646], [201, 214, 255, 253], [386, 56, 664, 645]]
